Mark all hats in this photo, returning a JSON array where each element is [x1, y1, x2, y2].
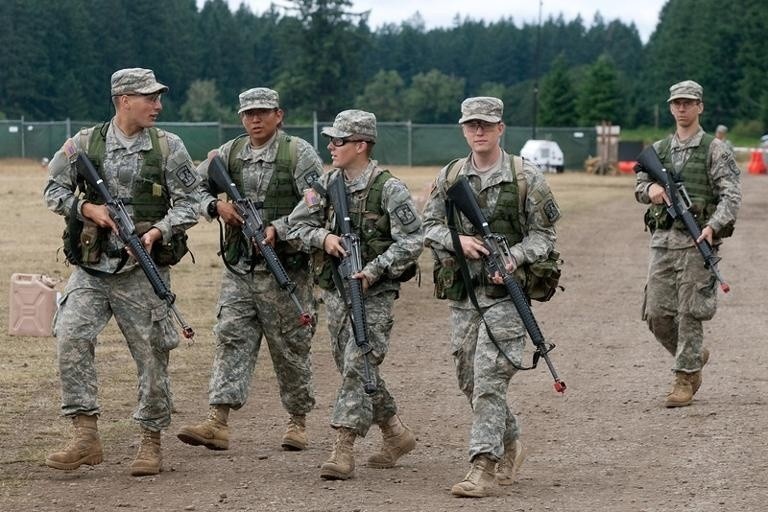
[[667, 81, 703, 102], [110, 68, 169, 96], [459, 97, 504, 124], [321, 109, 377, 138], [237, 88, 279, 114]]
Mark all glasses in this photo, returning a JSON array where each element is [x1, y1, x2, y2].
[[464, 122, 495, 131], [329, 137, 367, 146]]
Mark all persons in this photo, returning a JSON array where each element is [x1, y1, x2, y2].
[[176, 87, 325, 451], [285, 109, 426, 480], [634, 80, 742, 408], [42, 68, 201, 477], [420, 97, 563, 498], [716, 125, 736, 158]]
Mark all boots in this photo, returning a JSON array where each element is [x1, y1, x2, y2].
[[320, 428, 356, 478], [46, 416, 104, 470], [282, 414, 306, 450], [665, 347, 709, 407], [496, 441, 525, 485], [176, 404, 229, 449], [451, 455, 504, 497], [367, 414, 416, 469], [130, 430, 163, 474]]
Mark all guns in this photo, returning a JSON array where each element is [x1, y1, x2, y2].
[[638, 145, 729, 292], [207, 155, 311, 326], [75, 150, 193, 340], [448, 175, 565, 395], [326, 174, 376, 393]]
